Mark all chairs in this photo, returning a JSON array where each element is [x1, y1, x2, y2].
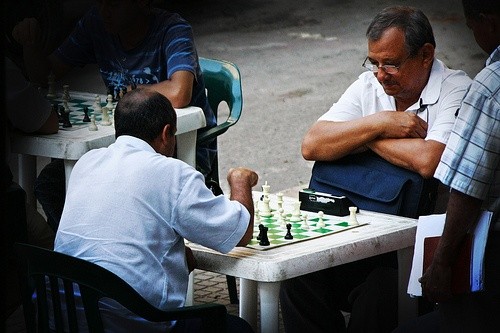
[[197, 57, 243, 196], [14, 241, 227, 333]]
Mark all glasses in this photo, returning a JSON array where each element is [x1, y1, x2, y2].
[[362, 42, 425, 75]]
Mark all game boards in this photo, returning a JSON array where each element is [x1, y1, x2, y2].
[[41, 91, 117, 132], [245, 201, 369, 252]]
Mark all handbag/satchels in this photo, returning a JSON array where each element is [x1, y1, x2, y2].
[[308, 151, 432, 219]]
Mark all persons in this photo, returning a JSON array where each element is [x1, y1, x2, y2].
[[30, 87, 259, 333], [10, 0, 217, 232], [418, 0, 500, 333], [279, 4, 473, 333], [0, 0, 59, 333]]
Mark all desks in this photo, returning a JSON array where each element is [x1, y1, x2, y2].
[[182, 191, 417, 333], [12, 90, 206, 199]]
